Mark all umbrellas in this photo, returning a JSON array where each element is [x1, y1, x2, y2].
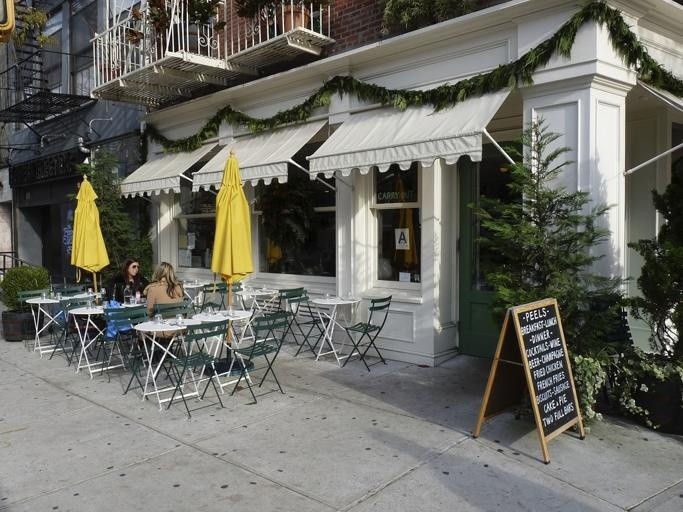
[[69, 173, 110, 303], [390, 178, 420, 272]]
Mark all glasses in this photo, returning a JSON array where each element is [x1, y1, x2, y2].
[[129, 264, 140, 269]]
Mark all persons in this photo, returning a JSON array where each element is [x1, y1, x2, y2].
[[106, 257, 150, 335], [135, 261, 184, 364], [263, 238, 282, 276], [208, 151, 255, 344]]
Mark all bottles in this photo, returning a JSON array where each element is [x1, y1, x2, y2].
[[123, 284, 131, 305], [48, 283, 54, 299], [85, 287, 95, 310]]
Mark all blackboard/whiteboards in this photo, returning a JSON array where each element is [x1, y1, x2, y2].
[[513, 299, 581, 441]]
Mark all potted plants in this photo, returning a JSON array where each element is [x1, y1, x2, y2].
[[1, 264, 50, 341], [231, 0, 334, 35]]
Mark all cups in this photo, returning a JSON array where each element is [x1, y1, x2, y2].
[[40, 292, 47, 299], [55, 292, 62, 300], [129, 298, 136, 305], [154, 313, 163, 323], [140, 298, 146, 304], [174, 313, 184, 325], [226, 305, 235, 316], [206, 306, 213, 316], [193, 279, 198, 285]]
